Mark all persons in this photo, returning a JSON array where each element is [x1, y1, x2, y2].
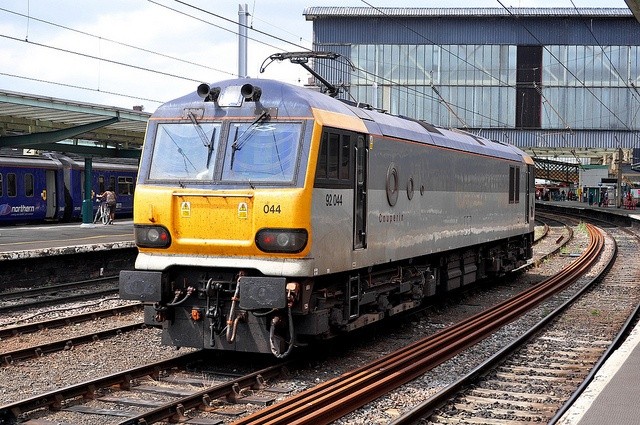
[[544, 192, 548, 201], [561, 190, 565, 201], [623, 190, 627, 208], [589, 191, 593, 205], [604, 191, 608, 207], [568, 189, 572, 201], [100, 185, 117, 225], [538, 189, 542, 200], [627, 190, 633, 208], [599, 189, 605, 207]]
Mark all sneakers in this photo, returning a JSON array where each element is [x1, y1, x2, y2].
[[108, 218, 113, 225]]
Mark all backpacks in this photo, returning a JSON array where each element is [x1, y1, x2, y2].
[[107, 192, 116, 204]]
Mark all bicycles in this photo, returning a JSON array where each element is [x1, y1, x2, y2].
[[92, 199, 112, 225]]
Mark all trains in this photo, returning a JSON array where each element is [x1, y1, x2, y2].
[[0, 150, 140, 225], [116, 76, 537, 362]]
